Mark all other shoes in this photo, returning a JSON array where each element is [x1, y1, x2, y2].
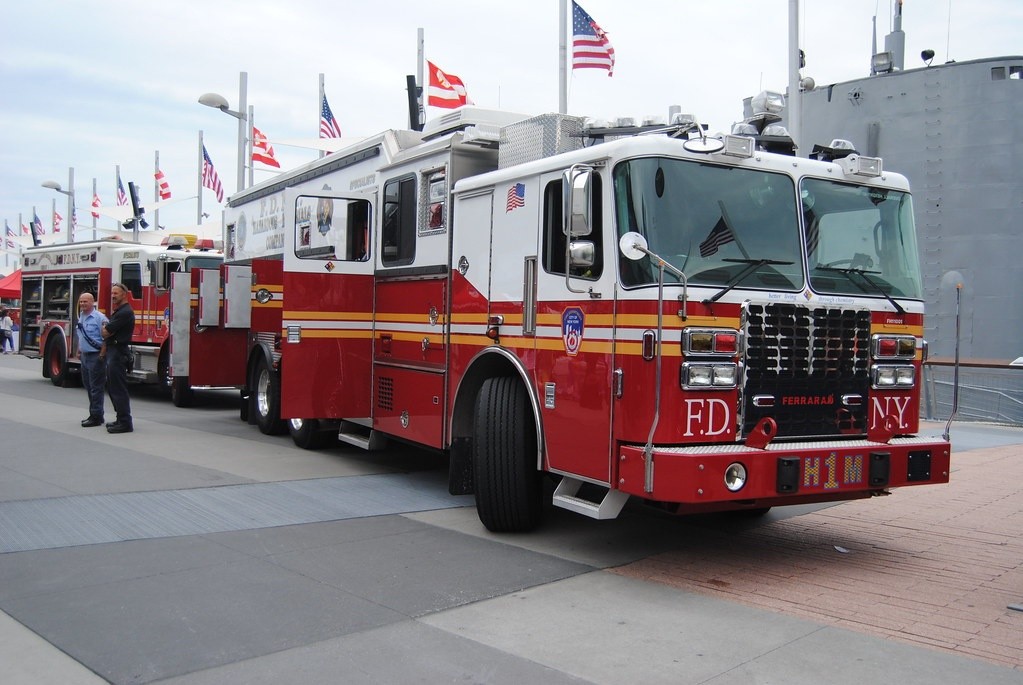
[[82, 416, 104, 423], [13, 352, 18, 354], [81, 417, 101, 426], [106, 421, 117, 427], [107, 424, 132, 433], [3, 351, 8, 354]]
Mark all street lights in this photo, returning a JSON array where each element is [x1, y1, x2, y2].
[[198, 92, 247, 189], [41, 180, 75, 242]]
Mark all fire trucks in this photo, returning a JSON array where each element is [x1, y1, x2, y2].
[[18, 229, 253, 408], [221, 95, 960, 537]]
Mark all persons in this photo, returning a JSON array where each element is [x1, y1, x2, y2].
[[101, 283, 133, 433], [75, 293, 109, 427], [0, 305, 16, 354]]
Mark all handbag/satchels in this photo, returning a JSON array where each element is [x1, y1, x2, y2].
[[4, 330, 12, 337]]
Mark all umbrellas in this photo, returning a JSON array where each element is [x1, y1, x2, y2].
[[0, 268, 22, 299]]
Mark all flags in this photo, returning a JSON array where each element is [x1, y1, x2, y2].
[[320, 93, 342, 155], [202, 144, 224, 202], [154, 170, 172, 200], [91, 193, 102, 218], [34, 213, 45, 235], [54, 213, 64, 232], [803, 202, 819, 258], [572, 0, 615, 77], [7, 226, 17, 248], [699, 216, 734, 258], [427, 61, 467, 109], [252, 126, 281, 168], [117, 177, 128, 206], [22, 224, 31, 235], [72, 199, 78, 242]]
